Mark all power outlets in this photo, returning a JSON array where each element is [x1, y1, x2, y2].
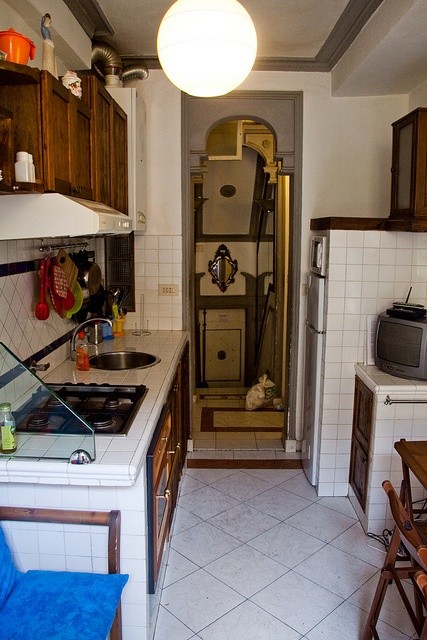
[[158, 285, 180, 294]]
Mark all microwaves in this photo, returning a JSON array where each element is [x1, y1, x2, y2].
[[309, 236, 327, 277]]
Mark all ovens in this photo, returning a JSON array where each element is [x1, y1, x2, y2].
[[145, 401, 179, 595]]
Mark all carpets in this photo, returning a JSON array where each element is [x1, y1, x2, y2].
[[200, 407, 286, 431]]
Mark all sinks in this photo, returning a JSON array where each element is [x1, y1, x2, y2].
[[89, 350, 161, 372]]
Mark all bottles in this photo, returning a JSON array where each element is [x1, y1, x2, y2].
[[0, 402, 16, 454], [29, 153, 35, 182], [14, 151, 30, 182]]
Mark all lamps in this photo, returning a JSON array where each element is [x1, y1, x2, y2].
[[155, 1, 258, 98]]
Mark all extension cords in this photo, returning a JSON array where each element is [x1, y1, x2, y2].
[[387, 530, 404, 557]]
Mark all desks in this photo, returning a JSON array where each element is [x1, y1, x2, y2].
[[394, 437, 427, 542]]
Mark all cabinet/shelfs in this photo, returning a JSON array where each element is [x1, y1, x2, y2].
[[0, 60, 43, 193], [107, 86, 147, 232], [0, 400, 189, 595], [161, 359, 189, 475], [40, 69, 94, 200], [91, 75, 129, 216], [348, 375, 427, 514], [388, 106, 426, 221]]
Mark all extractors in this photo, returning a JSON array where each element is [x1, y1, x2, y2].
[[0, 192, 132, 241]]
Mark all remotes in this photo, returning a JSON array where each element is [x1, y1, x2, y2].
[[391, 300, 424, 310]]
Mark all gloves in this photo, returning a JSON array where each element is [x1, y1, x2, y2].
[[48, 256, 75, 316]]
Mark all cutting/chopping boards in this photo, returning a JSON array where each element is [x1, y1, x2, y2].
[[54, 248, 78, 290]]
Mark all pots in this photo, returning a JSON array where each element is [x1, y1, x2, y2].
[[80, 261, 101, 295]]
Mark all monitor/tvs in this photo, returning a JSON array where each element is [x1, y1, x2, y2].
[[373, 311, 426, 380]]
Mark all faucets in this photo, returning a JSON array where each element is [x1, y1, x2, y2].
[[70, 317, 113, 361]]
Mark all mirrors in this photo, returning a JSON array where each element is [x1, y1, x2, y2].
[[208, 242, 239, 293]]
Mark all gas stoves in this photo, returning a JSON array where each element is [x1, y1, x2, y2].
[[0, 381, 149, 436]]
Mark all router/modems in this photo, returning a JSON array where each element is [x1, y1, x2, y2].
[[386, 286, 425, 319]]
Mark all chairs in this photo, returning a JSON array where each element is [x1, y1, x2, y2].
[[363, 480, 427, 639], [0, 505, 129, 640]]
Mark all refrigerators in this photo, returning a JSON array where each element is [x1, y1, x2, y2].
[[299, 274, 326, 487]]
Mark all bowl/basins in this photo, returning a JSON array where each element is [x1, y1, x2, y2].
[[62, 280, 83, 319]]
